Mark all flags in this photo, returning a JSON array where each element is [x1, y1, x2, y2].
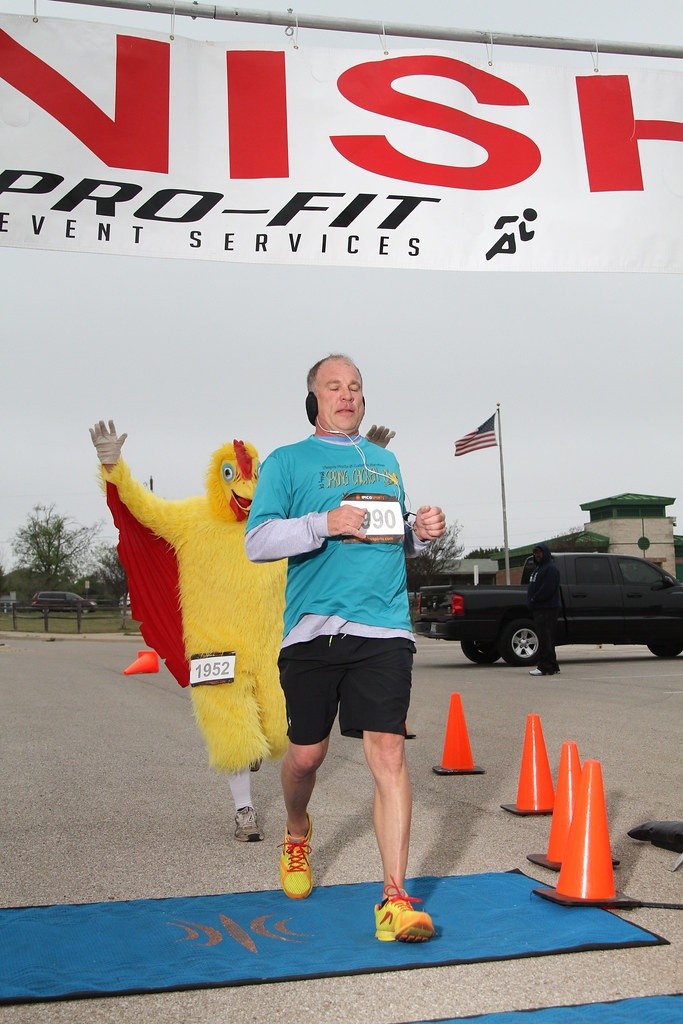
[[455, 413, 498, 457]]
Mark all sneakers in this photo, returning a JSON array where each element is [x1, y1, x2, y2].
[[375, 874, 436, 942], [528, 668, 544, 676], [234, 806, 264, 842], [250, 758, 262, 771], [277, 812, 312, 899]]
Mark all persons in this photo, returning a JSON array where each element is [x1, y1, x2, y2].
[[527, 544, 561, 676], [245, 355, 446, 942], [89, 419, 394, 843]]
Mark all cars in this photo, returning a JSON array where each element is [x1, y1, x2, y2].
[[118, 592, 131, 609]]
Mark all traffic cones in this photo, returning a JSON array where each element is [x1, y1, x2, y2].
[[431, 691, 487, 774], [533, 759, 644, 907], [526, 740, 622, 873], [500, 712, 554, 817], [124, 650, 160, 674]]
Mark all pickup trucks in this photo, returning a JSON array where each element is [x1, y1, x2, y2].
[[412, 551, 683, 666]]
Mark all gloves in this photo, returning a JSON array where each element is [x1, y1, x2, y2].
[[90, 420, 127, 465], [365, 425, 396, 449]]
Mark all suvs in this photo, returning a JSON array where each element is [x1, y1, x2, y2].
[[32, 590, 99, 614]]
